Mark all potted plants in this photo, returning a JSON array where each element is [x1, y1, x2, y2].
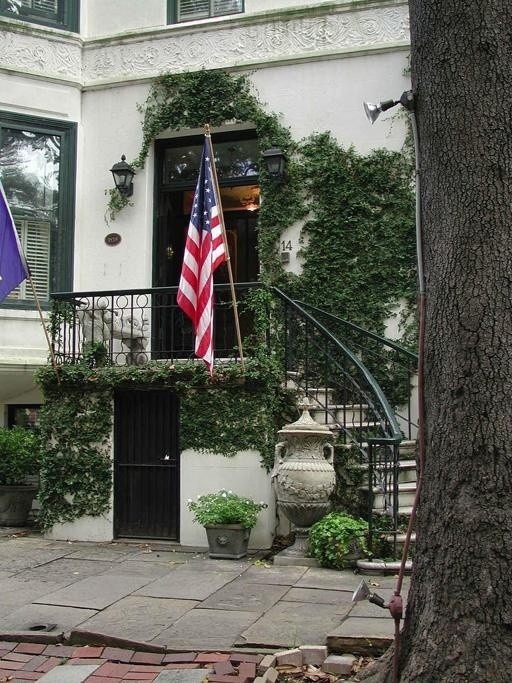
[[304, 512, 374, 570], [0, 425, 43, 525]]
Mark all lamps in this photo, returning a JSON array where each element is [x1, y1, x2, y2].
[[262, 146, 288, 186], [110, 154, 136, 197], [350, 579, 391, 609], [363, 90, 414, 125]]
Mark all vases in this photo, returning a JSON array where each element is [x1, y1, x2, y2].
[[203, 523, 252, 559]]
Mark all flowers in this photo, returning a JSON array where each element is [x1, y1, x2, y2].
[[186, 488, 268, 529]]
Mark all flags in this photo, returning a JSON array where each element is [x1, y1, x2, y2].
[[0, 188, 32, 309], [174, 134, 231, 384]]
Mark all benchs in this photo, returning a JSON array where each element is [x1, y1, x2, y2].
[[77, 304, 150, 365]]
